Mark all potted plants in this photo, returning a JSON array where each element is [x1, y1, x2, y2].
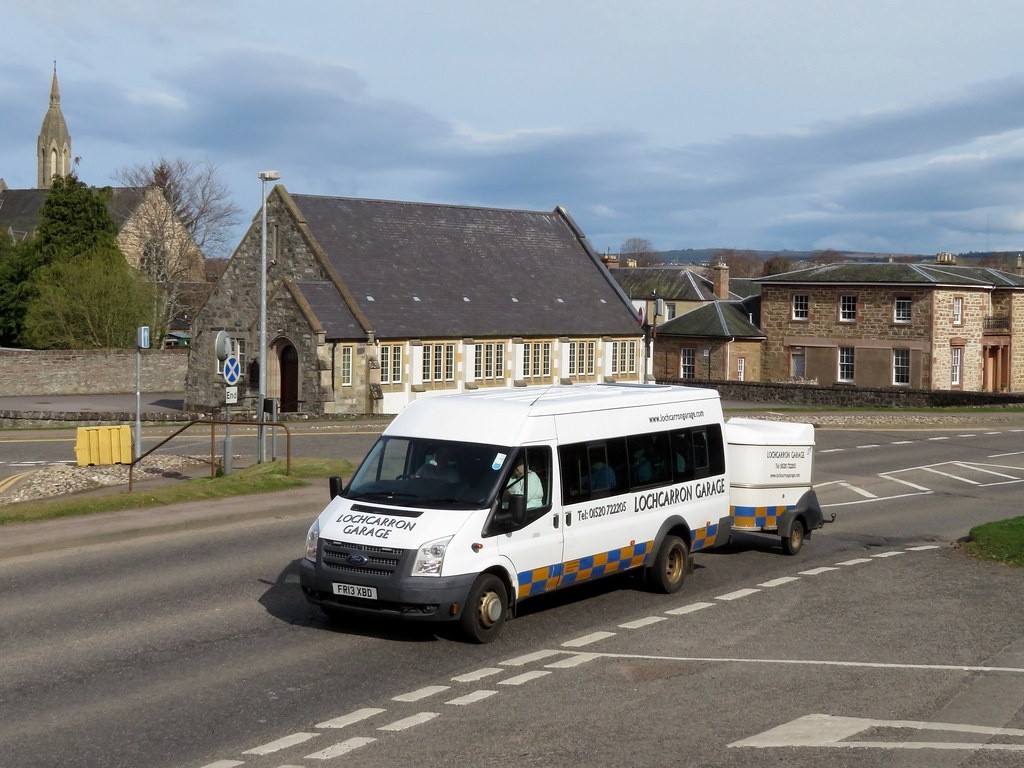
[[1001, 383, 1008, 392], [982, 385, 987, 392]]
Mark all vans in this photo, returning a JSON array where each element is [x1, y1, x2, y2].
[[299, 382, 732, 643]]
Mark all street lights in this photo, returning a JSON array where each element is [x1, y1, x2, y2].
[[257, 171, 280, 463]]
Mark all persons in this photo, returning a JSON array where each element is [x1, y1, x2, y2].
[[630, 447, 653, 482], [661, 445, 686, 473], [496, 459, 543, 508], [585, 453, 617, 490]]
